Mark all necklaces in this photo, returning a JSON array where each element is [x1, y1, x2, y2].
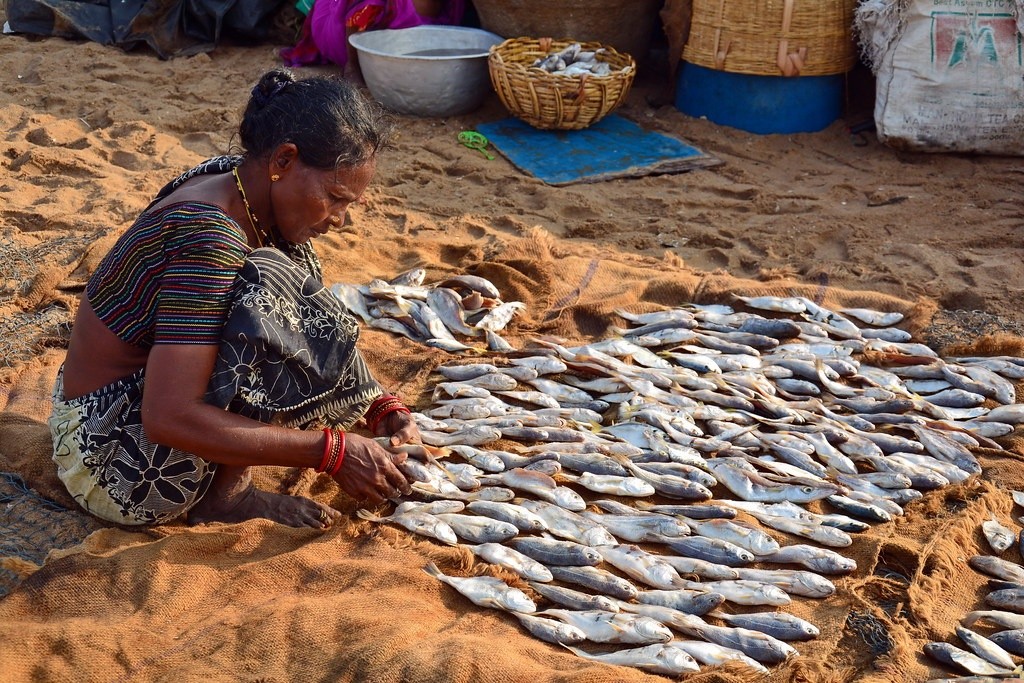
[[232, 166, 278, 249]]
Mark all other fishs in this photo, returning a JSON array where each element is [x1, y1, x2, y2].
[[332, 267, 1024, 679], [534, 43, 613, 77]]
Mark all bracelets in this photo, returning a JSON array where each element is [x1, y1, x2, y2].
[[316, 428, 346, 476], [365, 396, 410, 435]]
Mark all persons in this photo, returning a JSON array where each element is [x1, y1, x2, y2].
[[48, 67, 428, 529]]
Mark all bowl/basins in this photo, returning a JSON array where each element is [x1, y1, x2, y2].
[[348, 24, 506, 116]]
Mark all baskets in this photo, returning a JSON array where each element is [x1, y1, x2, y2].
[[489, 37, 636, 130]]
